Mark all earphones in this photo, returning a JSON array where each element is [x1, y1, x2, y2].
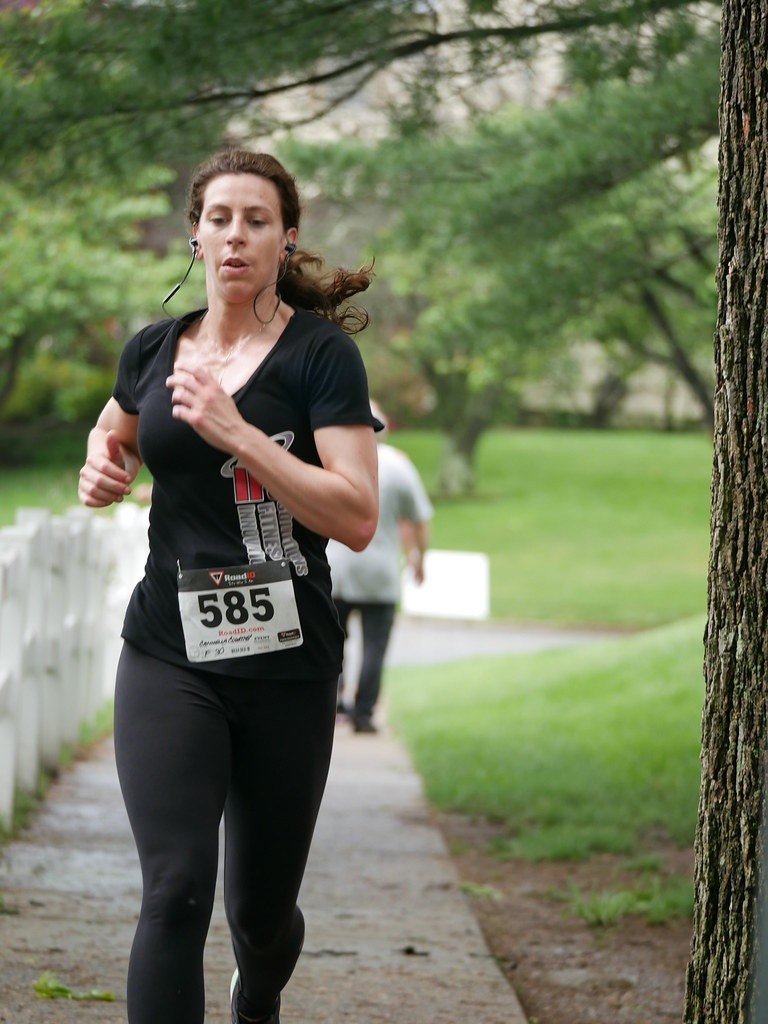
[[189, 237, 198, 254], [285, 242, 297, 259]]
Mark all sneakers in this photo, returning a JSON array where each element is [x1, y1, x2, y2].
[[230, 967, 281, 1023]]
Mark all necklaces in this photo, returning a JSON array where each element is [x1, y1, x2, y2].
[[204, 323, 268, 389]]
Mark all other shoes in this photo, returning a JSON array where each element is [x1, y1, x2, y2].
[[337, 703, 377, 733]]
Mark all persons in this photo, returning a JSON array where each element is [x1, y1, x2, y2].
[[77, 153, 391, 1024], [323, 397, 436, 733]]
